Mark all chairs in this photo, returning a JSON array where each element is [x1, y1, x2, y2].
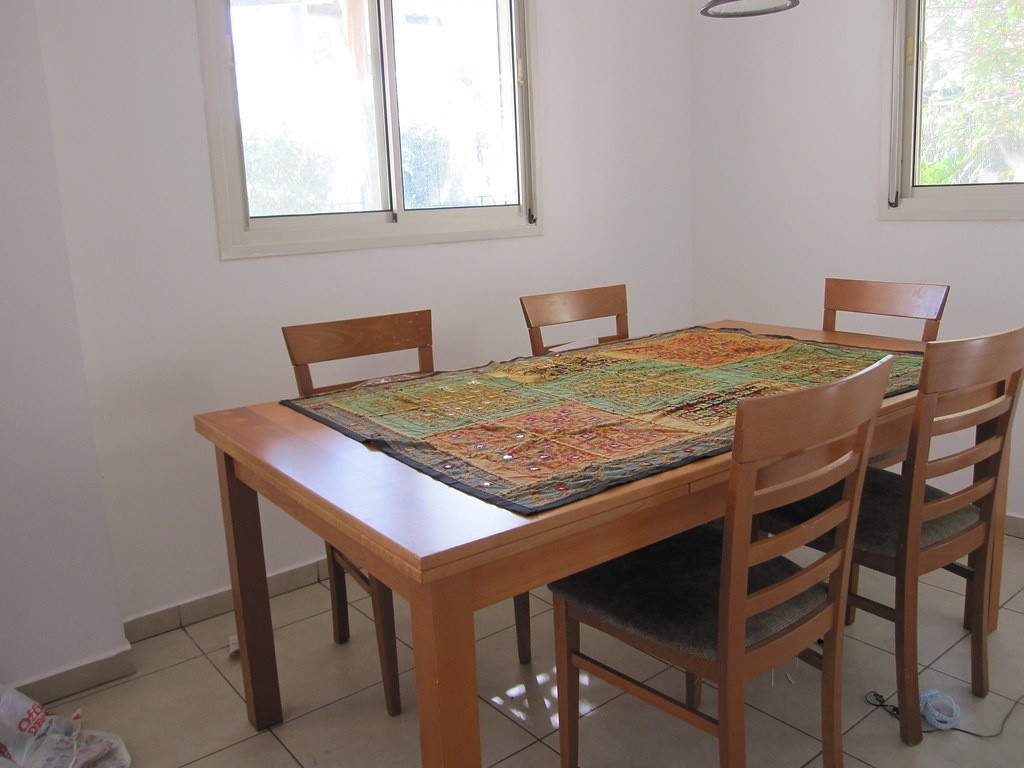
[[751, 328, 1024, 745], [804, 278, 950, 625], [521, 286, 630, 357], [546, 354, 897, 768], [281, 309, 531, 715]]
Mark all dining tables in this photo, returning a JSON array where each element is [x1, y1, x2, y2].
[[192, 320, 1015, 768]]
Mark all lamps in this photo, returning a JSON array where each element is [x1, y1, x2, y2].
[[700, 0, 800, 18]]
